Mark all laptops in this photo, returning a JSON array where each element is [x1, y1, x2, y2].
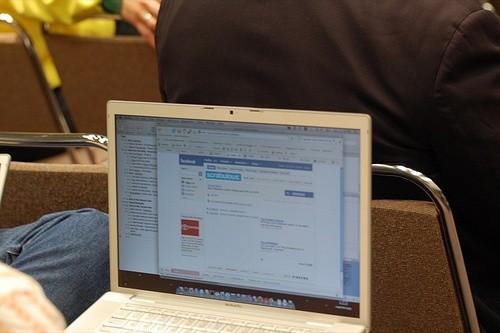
[[64, 99, 371, 333], [0, 154, 11, 203]]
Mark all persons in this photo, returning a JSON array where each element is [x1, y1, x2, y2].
[[0, 207, 110, 333], [0, 0, 161, 163], [155, 0, 500, 333]]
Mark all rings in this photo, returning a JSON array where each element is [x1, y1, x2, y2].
[[143, 13, 152, 21]]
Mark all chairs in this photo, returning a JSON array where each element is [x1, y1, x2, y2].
[[0, 6, 483, 333]]
